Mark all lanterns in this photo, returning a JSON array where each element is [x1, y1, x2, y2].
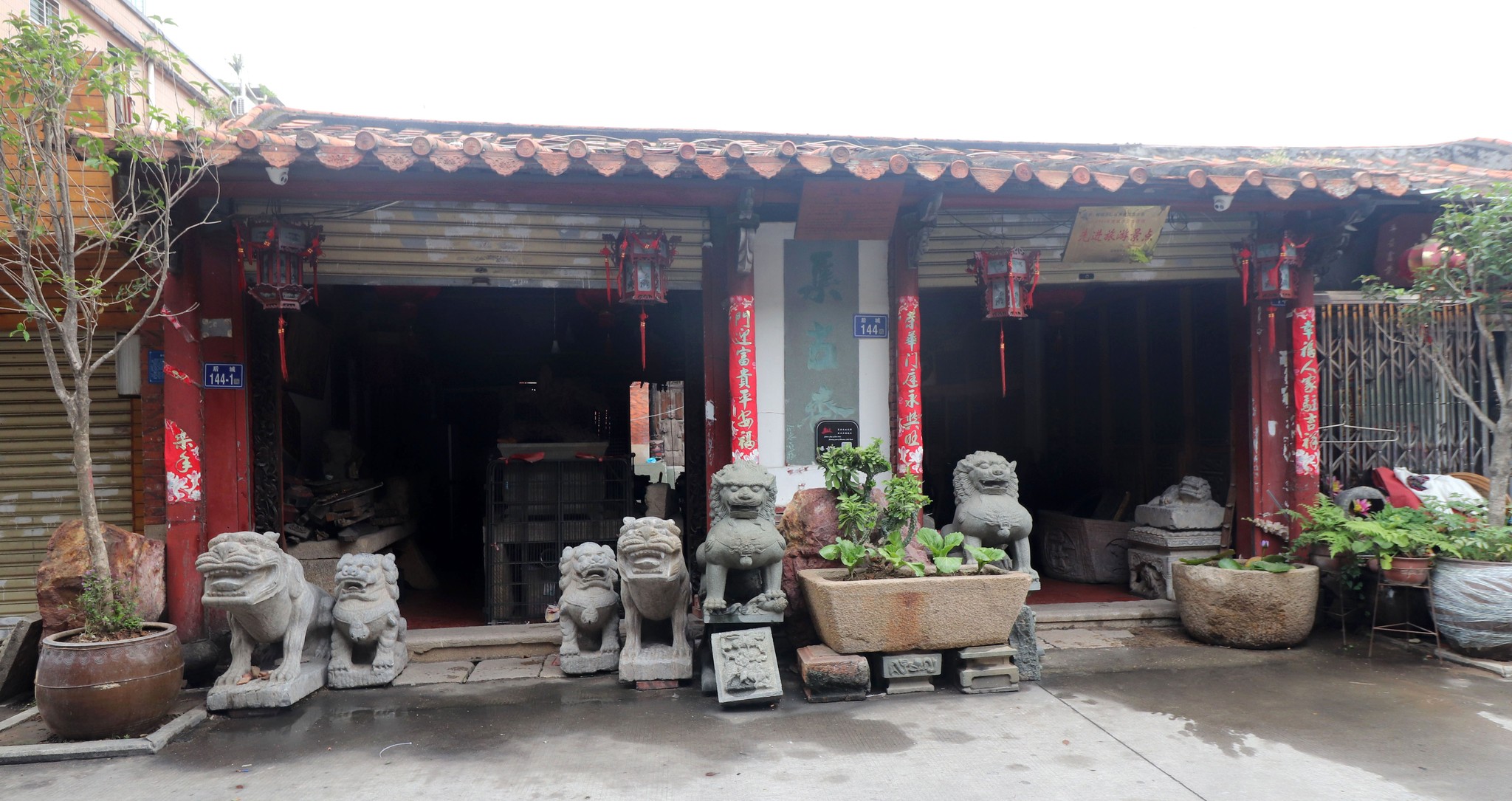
[[230, 213, 326, 381], [1398, 235, 1468, 292], [1229, 227, 1312, 354], [966, 245, 1041, 398], [601, 225, 682, 370]]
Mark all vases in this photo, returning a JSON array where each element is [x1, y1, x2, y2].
[[34, 621, 185, 741], [1171, 560, 1320, 650]]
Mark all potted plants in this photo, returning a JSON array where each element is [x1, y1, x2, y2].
[[797, 436, 1033, 654], [1038, 509, 1136, 584], [1274, 493, 1512, 661]]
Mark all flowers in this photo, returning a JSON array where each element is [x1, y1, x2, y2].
[[1321, 475, 1372, 518], [1178, 540, 1302, 573], [58, 567, 145, 638]]
